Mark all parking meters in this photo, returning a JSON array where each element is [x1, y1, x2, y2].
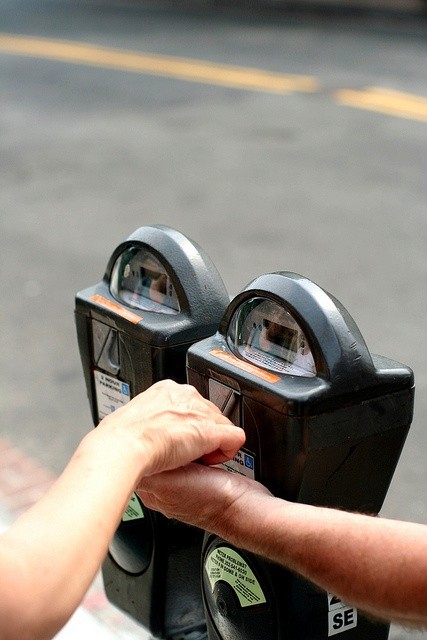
[[73, 223, 414, 640]]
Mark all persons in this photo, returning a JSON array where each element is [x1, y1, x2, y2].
[[0, 376, 248, 640], [134, 460, 426, 631]]
